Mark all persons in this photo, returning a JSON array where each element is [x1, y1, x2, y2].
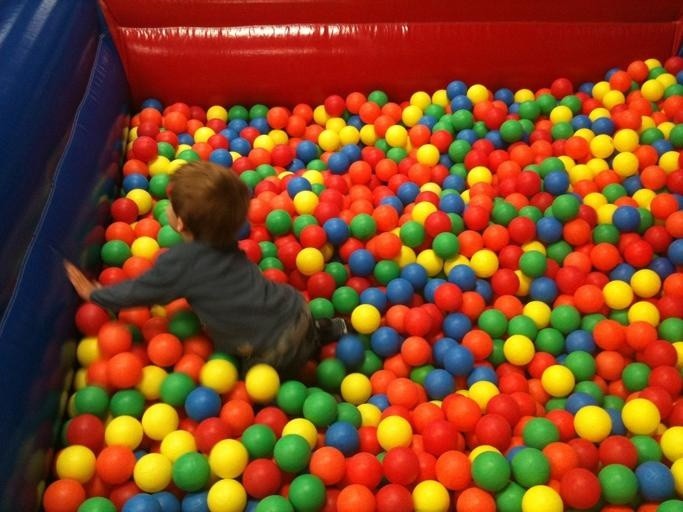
[[63, 163, 347, 386]]
